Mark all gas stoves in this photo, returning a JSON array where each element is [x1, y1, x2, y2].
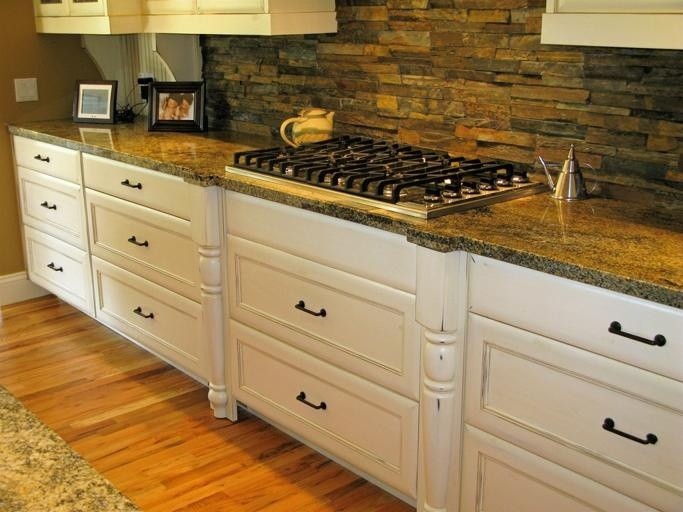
[[226, 133, 552, 221]]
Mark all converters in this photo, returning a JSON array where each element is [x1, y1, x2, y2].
[[138, 78, 153, 84], [141, 86, 148, 99]]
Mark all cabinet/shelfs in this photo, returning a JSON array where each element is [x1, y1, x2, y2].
[[8, 134, 682, 512], [540, 0, 683, 50], [33, 0, 337, 36]]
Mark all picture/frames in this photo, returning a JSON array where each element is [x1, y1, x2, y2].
[[72, 79, 118, 124], [148, 81, 207, 132]]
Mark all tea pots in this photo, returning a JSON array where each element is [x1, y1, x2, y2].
[[279, 105, 337, 146], [536, 143, 600, 202]]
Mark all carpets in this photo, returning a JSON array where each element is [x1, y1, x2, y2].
[[1, 388, 147, 512]]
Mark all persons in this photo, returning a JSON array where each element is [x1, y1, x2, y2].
[[160, 93, 182, 120], [175, 94, 193, 120]]
